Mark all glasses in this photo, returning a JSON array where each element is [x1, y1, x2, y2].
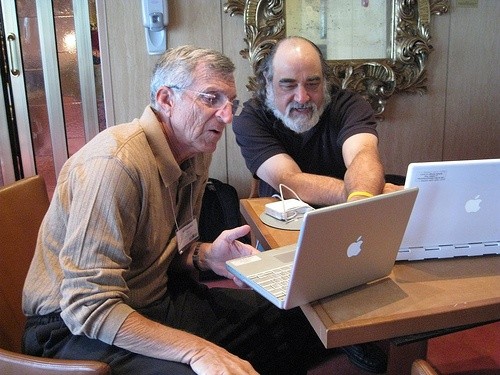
[[169, 86, 245, 117]]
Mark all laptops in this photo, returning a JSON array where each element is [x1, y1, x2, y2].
[[226, 186, 420, 310], [395, 158, 500, 261]]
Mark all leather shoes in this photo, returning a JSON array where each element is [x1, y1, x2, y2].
[[338, 342, 387, 374]]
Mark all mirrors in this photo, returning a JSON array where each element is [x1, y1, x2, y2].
[[222, 0, 451, 122]]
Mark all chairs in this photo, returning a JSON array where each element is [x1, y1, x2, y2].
[[0, 176, 111, 375]]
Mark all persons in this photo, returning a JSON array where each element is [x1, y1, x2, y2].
[[232, 37, 404, 372], [21, 45, 334, 375]]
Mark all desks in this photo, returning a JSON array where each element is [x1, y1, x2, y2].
[[239, 197, 500, 375]]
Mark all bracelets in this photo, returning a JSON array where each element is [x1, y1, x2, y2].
[[347, 192, 374, 202], [193, 242, 205, 272]]
